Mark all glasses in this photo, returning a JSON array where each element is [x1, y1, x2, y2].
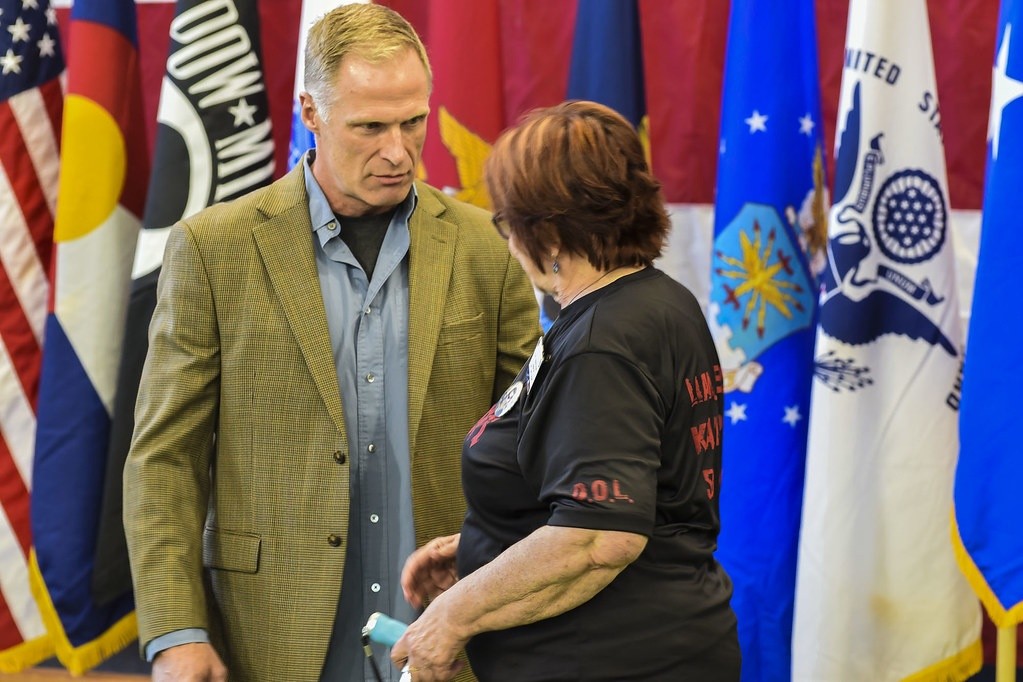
[[491, 211, 515, 239]]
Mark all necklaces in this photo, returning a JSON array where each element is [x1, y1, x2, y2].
[[566, 264, 627, 307]]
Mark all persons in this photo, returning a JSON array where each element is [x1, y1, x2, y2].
[[390, 98, 741, 682], [126, 2, 536, 682]]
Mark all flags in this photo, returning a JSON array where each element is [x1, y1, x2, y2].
[[0, 0, 1023, 682]]
